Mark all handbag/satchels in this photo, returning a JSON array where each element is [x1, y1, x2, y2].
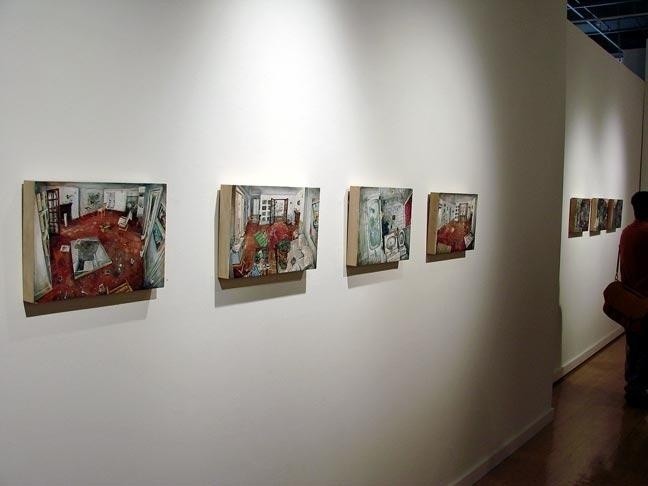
[[603, 280, 647, 336]]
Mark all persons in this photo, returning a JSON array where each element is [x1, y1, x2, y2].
[[619, 190, 647, 412]]
